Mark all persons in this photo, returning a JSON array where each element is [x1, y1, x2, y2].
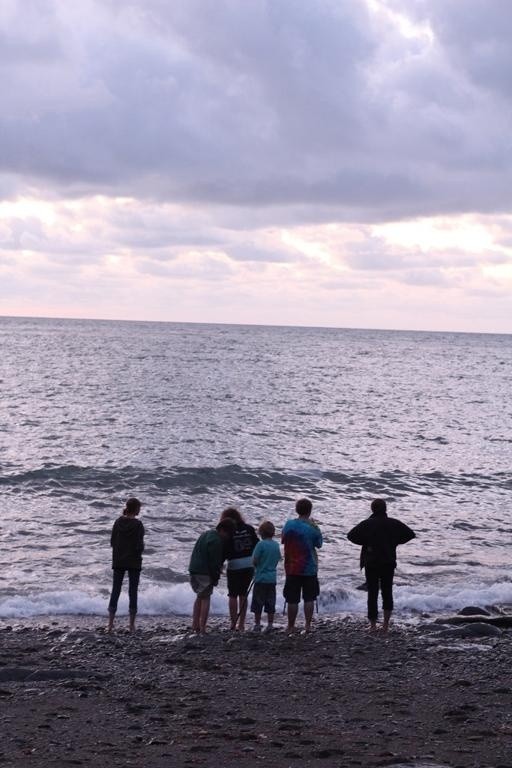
[[249, 519, 283, 635], [279, 497, 325, 636], [103, 498, 146, 633], [346, 499, 416, 635], [187, 517, 237, 638], [218, 507, 261, 633]]
